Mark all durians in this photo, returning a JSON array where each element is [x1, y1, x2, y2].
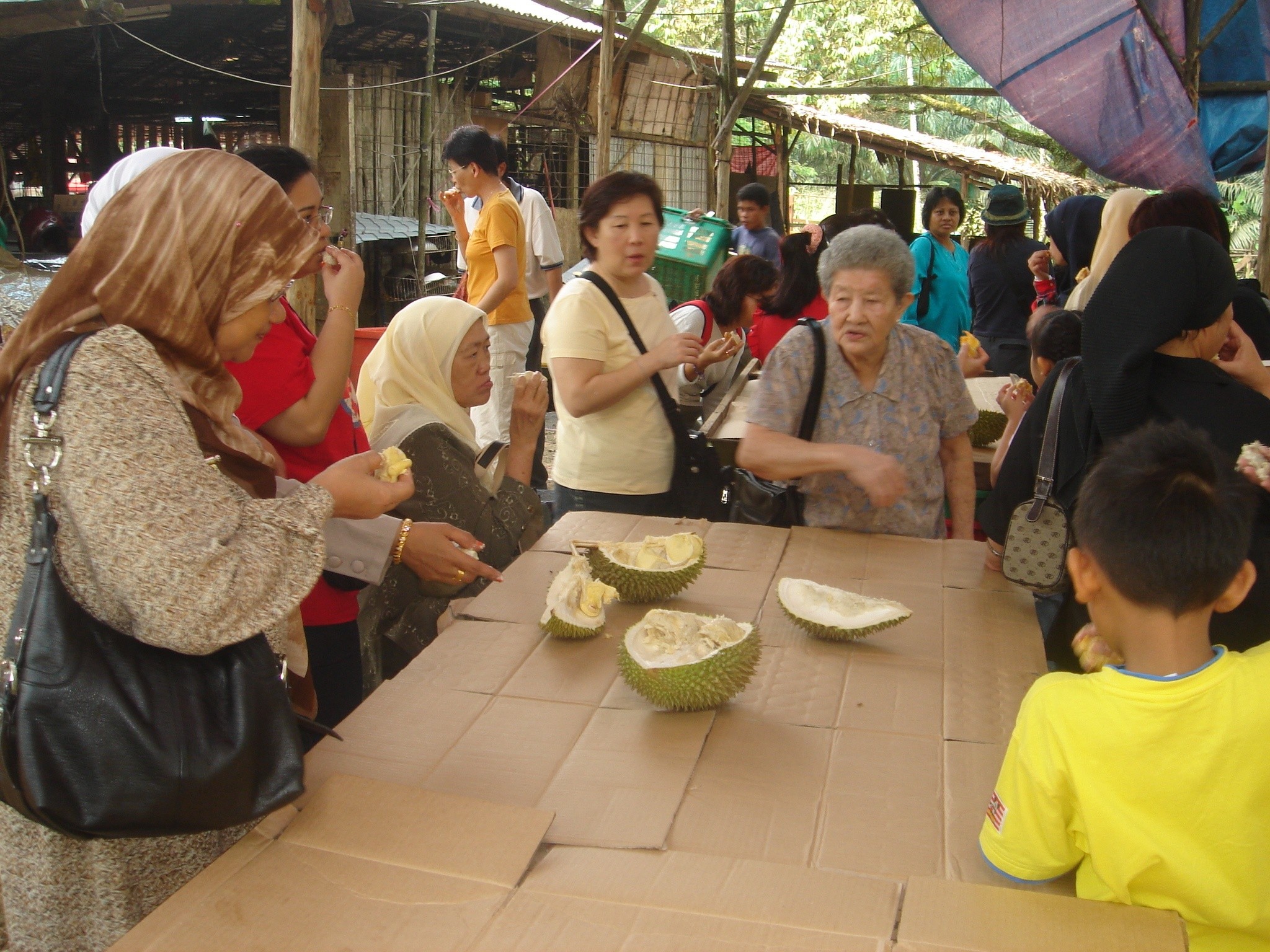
[[511, 370, 535, 386], [967, 411, 1007, 445], [573, 531, 708, 603], [621, 606, 764, 711], [538, 557, 621, 639], [371, 446, 412, 482]]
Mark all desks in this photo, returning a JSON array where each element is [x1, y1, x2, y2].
[[692, 355, 1024, 466]]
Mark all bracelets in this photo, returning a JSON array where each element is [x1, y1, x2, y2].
[[327, 305, 356, 326], [393, 518, 412, 565], [693, 365, 705, 382]]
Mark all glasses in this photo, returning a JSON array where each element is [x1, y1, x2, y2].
[[270, 279, 295, 302], [1044, 227, 1051, 237], [447, 163, 481, 179], [304, 206, 334, 227]]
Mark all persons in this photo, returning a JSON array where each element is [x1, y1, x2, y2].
[[356, 296, 549, 658], [736, 224, 979, 540], [689, 182, 781, 268], [900, 186, 972, 354], [540, 172, 703, 516], [968, 184, 1048, 384], [438, 125, 535, 449], [0, 146, 504, 952], [989, 187, 1270, 673], [977, 423, 1270, 952], [456, 136, 564, 372], [671, 254, 780, 424], [751, 208, 897, 367], [220, 144, 370, 747]]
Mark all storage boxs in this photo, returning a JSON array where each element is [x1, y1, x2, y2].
[[647, 205, 731, 304], [53, 193, 87, 250]]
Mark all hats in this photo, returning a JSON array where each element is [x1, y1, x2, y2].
[[980, 184, 1031, 225]]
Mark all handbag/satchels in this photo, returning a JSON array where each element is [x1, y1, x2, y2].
[[916, 236, 937, 318], [0, 330, 344, 839], [719, 466, 804, 528], [1002, 356, 1083, 596], [674, 429, 724, 520]]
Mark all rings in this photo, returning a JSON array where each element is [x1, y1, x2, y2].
[[453, 570, 465, 581], [726, 351, 730, 356]]
